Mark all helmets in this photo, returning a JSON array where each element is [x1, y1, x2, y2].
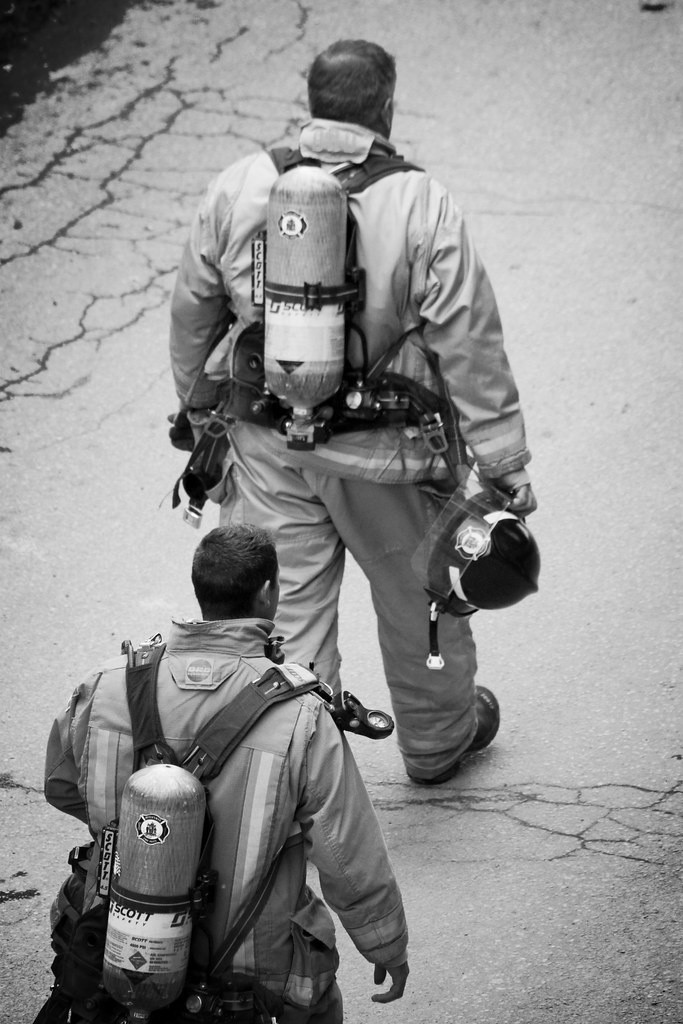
[[424, 485, 542, 618]]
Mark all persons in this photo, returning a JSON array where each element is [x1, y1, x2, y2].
[[44, 524, 410, 1024], [171, 39, 538, 784]]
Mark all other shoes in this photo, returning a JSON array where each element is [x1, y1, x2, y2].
[[406, 685, 500, 784]]
